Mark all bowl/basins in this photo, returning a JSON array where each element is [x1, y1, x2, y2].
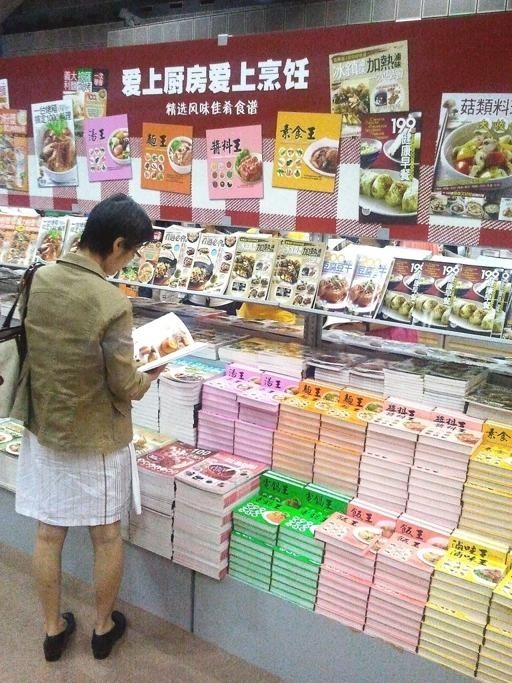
[[387, 270, 512, 304], [43, 164, 77, 184], [136, 261, 154, 284], [167, 135, 192, 176], [440, 121, 512, 192], [360, 136, 383, 166], [233, 151, 263, 185], [107, 127, 130, 165], [207, 464, 236, 481]]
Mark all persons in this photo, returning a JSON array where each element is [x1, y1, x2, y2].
[[10, 192, 170, 660]]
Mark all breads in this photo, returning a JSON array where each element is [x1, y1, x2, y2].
[[359, 171, 417, 213], [384, 294, 505, 333]]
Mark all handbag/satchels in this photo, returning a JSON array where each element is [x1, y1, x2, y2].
[[0, 325, 31, 417]]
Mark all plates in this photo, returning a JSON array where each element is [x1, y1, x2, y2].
[[473, 564, 503, 587], [380, 289, 496, 333], [415, 546, 445, 568], [382, 138, 420, 167], [261, 510, 282, 527], [171, 370, 204, 384], [0, 431, 21, 456], [359, 166, 420, 217], [301, 137, 340, 177], [353, 525, 383, 545]]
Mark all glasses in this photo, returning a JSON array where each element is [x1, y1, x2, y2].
[[132, 247, 143, 260]]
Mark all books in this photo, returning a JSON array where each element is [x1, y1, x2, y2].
[[240, 579, 512, 682], [1, 206, 511, 341], [0, 296, 511, 579]]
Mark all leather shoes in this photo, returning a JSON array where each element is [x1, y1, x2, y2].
[[44, 612, 75, 661], [91, 610, 126, 659]]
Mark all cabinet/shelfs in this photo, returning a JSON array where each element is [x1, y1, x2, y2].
[[0, 205, 511, 681]]
